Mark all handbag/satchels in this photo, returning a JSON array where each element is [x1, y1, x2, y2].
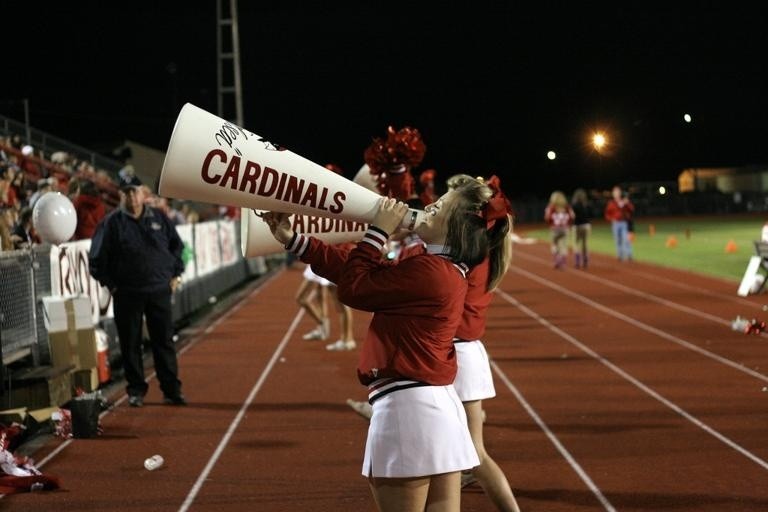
[[627, 220, 634, 232]]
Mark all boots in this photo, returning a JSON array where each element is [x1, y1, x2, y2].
[[552, 253, 590, 272]]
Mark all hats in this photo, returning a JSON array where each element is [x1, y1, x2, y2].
[[119, 173, 144, 191]]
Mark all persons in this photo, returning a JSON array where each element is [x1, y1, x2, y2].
[[200, 205, 238, 221], [571, 190, 593, 269], [604, 186, 635, 261], [544, 189, 576, 270], [296, 265, 356, 350], [347, 215, 522, 512], [89, 176, 185, 407], [0, 134, 199, 251], [261, 175, 522, 512]]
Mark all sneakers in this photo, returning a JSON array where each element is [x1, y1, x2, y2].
[[461, 474, 478, 489], [345, 398, 373, 419], [303, 319, 330, 341], [325, 340, 357, 352], [163, 392, 184, 404], [128, 395, 144, 407]]
